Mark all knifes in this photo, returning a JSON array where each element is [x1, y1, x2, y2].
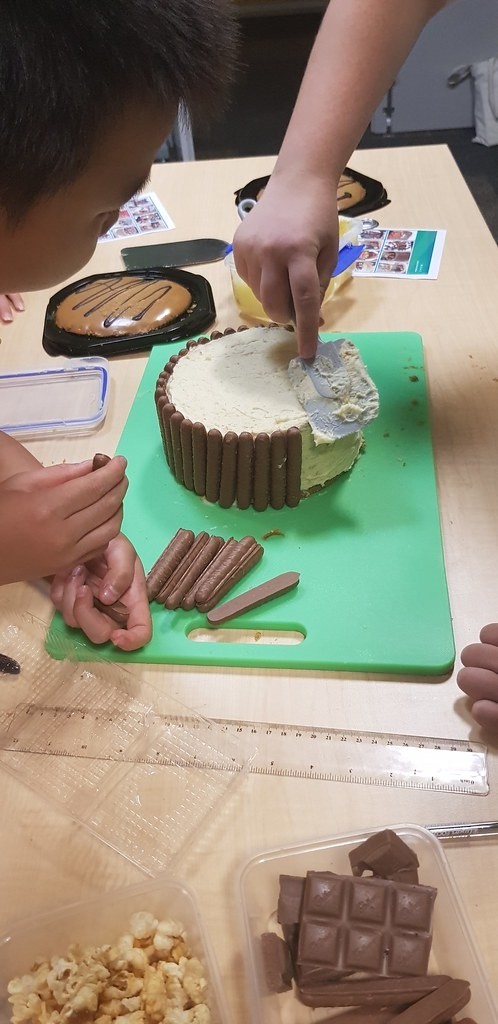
[[288, 336, 379, 444], [119, 236, 232, 270]]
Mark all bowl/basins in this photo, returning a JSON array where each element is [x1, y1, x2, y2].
[[0, 877, 234, 1024], [226, 822, 498, 1024], [226, 216, 363, 324]]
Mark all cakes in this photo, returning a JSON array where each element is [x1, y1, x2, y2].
[[152, 323, 363, 512]]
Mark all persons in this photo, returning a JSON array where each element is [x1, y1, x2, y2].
[[232, 0, 453, 361], [2, 0, 251, 651]]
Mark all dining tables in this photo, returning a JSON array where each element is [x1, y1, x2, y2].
[[1, 143, 497, 1024]]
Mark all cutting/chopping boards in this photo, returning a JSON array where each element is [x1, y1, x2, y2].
[[43, 328, 458, 677], [43, 265, 218, 360], [233, 170, 390, 221]]
